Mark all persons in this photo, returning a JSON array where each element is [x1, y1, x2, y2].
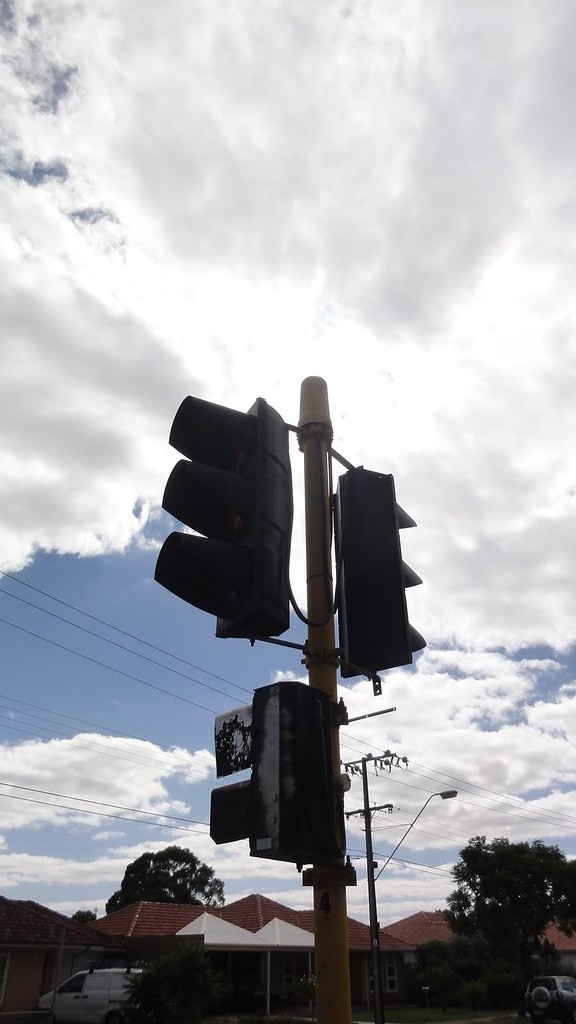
[[514, 973, 528, 1019], [532, 971, 544, 979]]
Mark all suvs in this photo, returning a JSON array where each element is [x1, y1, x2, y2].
[[524, 976, 576, 1024]]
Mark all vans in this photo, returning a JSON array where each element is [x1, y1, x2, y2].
[[31, 960, 144, 1024]]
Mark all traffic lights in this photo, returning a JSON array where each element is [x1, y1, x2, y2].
[[153, 395, 293, 640], [333, 466, 427, 678], [210, 682, 335, 863]]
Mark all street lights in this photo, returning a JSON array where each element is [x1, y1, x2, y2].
[[369, 789, 457, 1024]]
